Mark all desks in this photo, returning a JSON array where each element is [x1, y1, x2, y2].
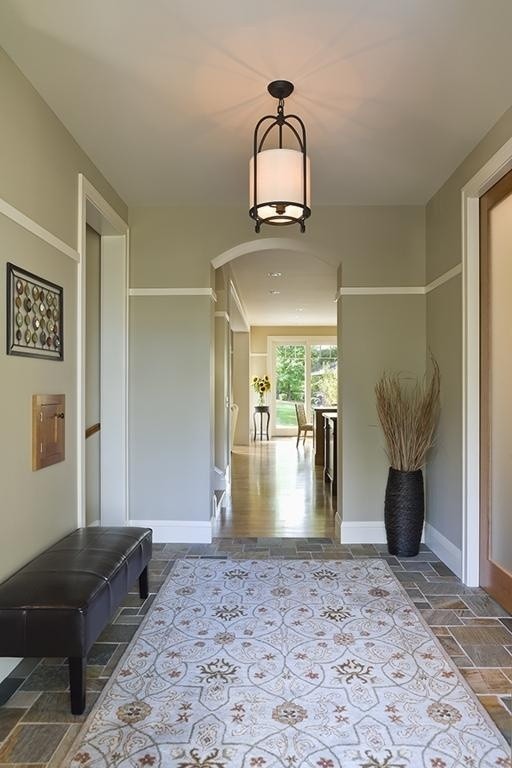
[[322, 412, 337, 497], [313, 407, 337, 466]]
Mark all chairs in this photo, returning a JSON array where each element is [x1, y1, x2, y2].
[[296, 404, 313, 448]]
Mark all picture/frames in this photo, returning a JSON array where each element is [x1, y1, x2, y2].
[[6, 261, 63, 362]]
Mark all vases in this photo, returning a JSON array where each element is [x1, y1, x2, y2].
[[385, 467, 424, 557], [257, 392, 265, 406]]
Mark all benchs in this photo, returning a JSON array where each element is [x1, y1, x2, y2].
[[0, 526, 154, 715]]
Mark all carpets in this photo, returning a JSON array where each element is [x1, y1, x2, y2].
[[57, 558, 512, 768]]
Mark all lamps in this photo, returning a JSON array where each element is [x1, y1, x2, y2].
[[248, 80, 312, 234]]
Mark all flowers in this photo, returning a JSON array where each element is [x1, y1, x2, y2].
[[252, 376, 271, 394]]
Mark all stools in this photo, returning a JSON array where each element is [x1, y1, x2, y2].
[[253, 406, 270, 441]]
